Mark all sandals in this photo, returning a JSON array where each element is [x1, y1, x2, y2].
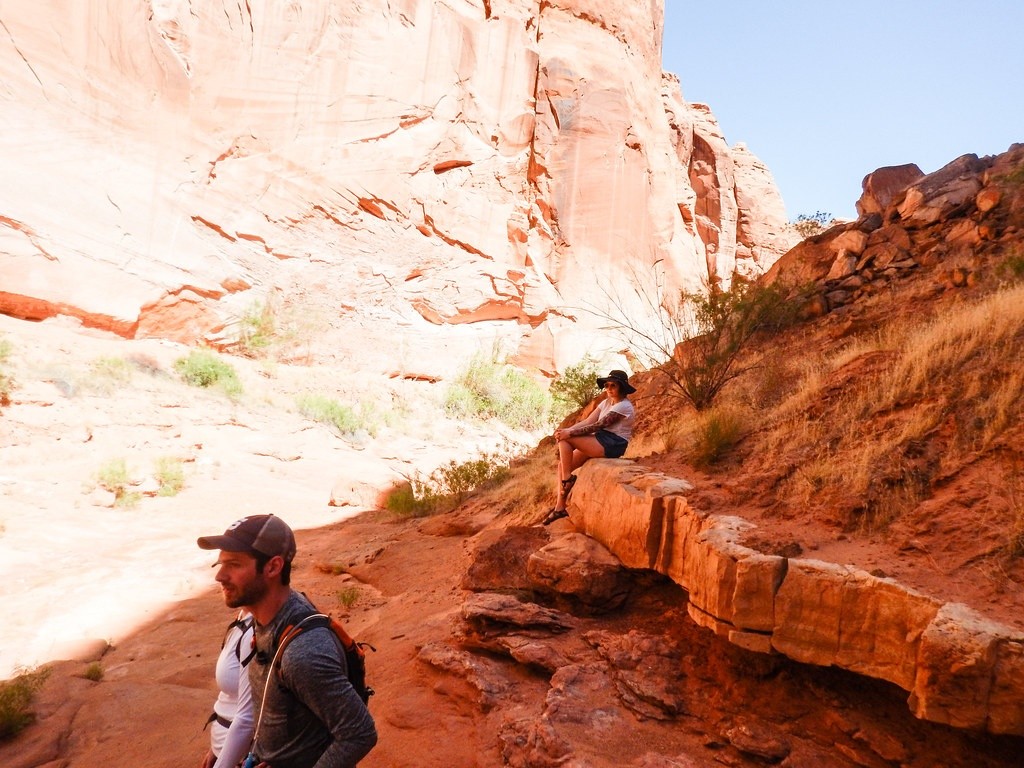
[[543, 510, 569, 525], [560, 474, 577, 498]]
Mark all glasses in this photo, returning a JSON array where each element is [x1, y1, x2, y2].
[[604, 383, 619, 388]]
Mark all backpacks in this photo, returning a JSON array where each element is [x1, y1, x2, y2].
[[240, 610, 376, 708]]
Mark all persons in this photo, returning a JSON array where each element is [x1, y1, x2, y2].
[[542, 370, 637, 526], [202, 607, 255, 768], [197, 513, 378, 768]]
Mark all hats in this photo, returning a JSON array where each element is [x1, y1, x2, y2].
[[197, 513, 296, 563], [597, 370, 636, 394]]
